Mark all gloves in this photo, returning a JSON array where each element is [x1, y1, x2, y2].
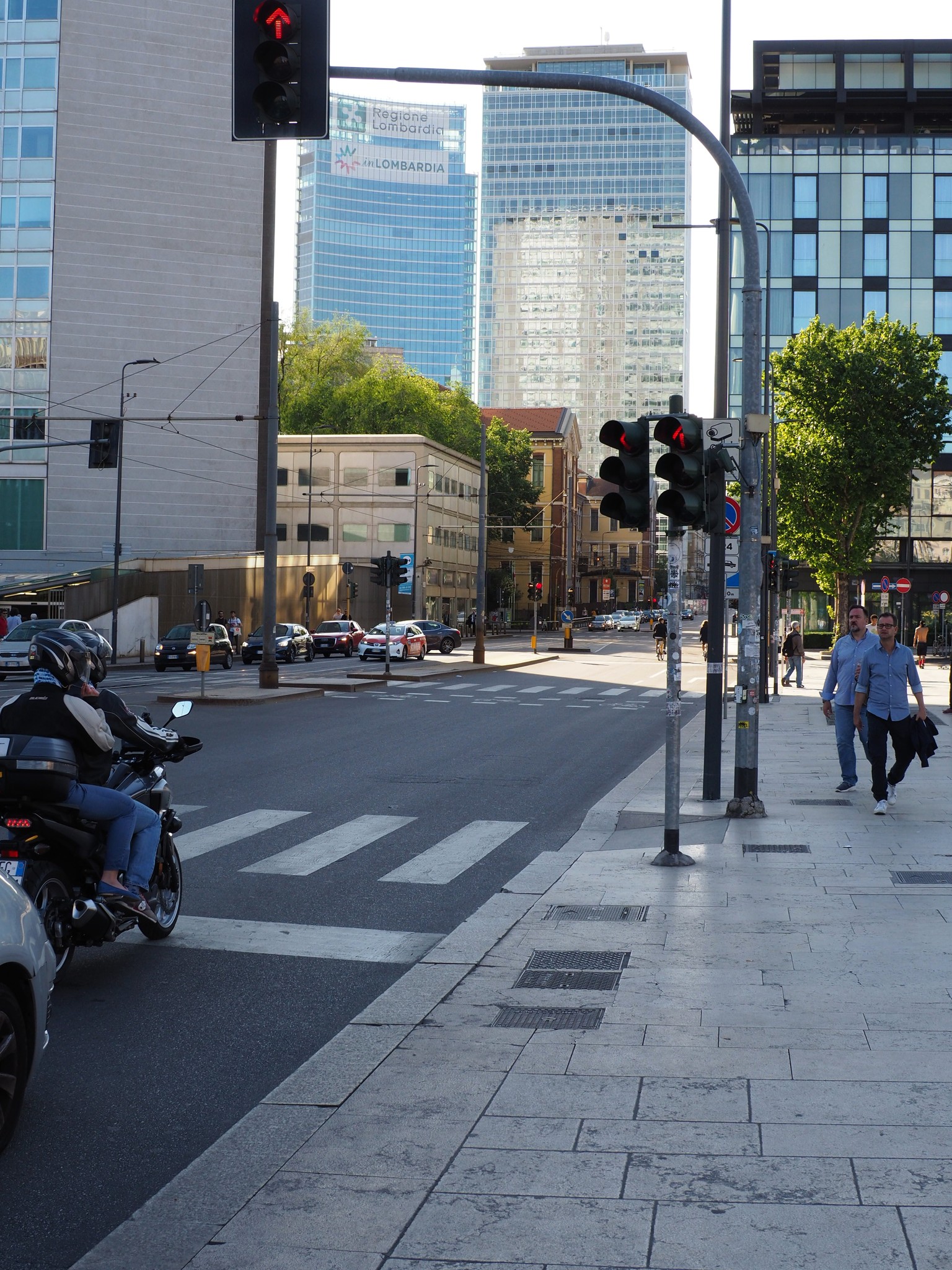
[[177, 735, 185, 745]]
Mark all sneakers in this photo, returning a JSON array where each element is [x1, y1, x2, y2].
[[886, 776, 897, 805], [835, 782, 856, 792], [655, 653, 658, 657], [114, 886, 157, 923], [781, 677, 786, 685], [797, 685, 804, 688], [662, 649, 666, 655], [921, 664, 924, 669], [919, 665, 921, 668], [97, 880, 140, 902], [874, 799, 888, 815]]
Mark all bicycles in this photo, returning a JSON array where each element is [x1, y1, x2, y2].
[[657, 636, 667, 661], [932, 635, 950, 657], [702, 642, 708, 661]]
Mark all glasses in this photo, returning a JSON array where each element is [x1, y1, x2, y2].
[[338, 611, 340, 611], [219, 613, 223, 614], [797, 626, 800, 627], [345, 611, 347, 612], [877, 624, 895, 629], [3, 613, 8, 614]]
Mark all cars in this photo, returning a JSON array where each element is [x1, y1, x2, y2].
[[0, 618, 92, 682], [0, 868, 57, 1156], [588, 608, 667, 632], [153, 619, 462, 672]]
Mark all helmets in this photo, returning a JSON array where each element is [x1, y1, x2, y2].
[[920, 621, 926, 625], [28, 628, 89, 690], [74, 629, 107, 685]]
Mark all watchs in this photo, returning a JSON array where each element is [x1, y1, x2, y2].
[[822, 699, 829, 703]]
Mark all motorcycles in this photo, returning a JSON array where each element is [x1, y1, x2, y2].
[[0, 692, 202, 985]]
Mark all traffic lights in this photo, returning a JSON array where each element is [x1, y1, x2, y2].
[[230, 0, 331, 143], [567, 588, 575, 605], [766, 553, 799, 592], [351, 583, 359, 598], [647, 598, 651, 607], [599, 416, 649, 529], [527, 581, 543, 601], [654, 416, 707, 526], [369, 556, 408, 586], [653, 598, 657, 607]]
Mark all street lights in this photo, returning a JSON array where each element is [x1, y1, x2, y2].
[[411, 464, 440, 621], [637, 542, 647, 608], [305, 425, 340, 637], [111, 360, 162, 664], [710, 217, 779, 697], [601, 531, 614, 614], [732, 357, 780, 678]]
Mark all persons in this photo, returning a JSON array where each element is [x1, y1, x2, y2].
[[227, 611, 243, 653], [582, 607, 588, 622], [332, 608, 343, 621], [0, 607, 22, 641], [782, 621, 806, 688], [653, 617, 667, 657], [819, 604, 880, 792], [591, 609, 596, 617], [624, 605, 644, 611], [443, 610, 449, 626], [596, 608, 599, 615], [341, 609, 353, 621], [215, 611, 228, 632], [29, 613, 37, 628], [913, 620, 929, 669], [67, 629, 183, 923], [0, 628, 144, 902], [699, 620, 708, 657], [467, 611, 476, 635], [942, 650, 952, 714], [601, 608, 606, 615], [853, 612, 927, 815], [866, 614, 878, 634]]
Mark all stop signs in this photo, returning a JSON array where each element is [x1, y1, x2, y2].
[[896, 578, 911, 593]]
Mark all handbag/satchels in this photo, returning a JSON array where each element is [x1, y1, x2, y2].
[[233, 624, 241, 636], [465, 615, 471, 628]]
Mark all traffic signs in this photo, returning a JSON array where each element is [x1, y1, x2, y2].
[[610, 589, 614, 598]]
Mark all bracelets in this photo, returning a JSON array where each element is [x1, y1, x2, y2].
[[802, 657, 804, 658], [913, 643, 915, 644]]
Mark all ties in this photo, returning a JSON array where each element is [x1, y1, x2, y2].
[[473, 615, 474, 622], [445, 615, 447, 621]]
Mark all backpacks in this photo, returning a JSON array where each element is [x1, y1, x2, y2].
[[782, 633, 801, 657]]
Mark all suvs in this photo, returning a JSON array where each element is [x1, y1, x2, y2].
[[682, 609, 694, 620]]
